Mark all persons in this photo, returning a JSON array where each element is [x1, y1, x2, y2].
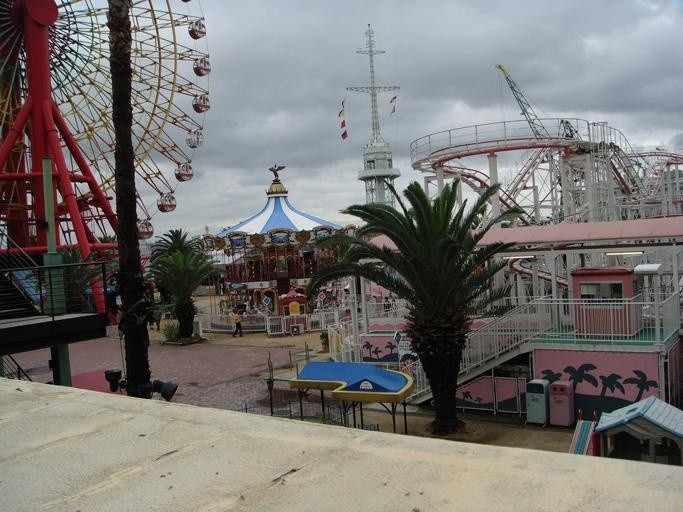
[[108, 307, 123, 325], [147, 305, 161, 331], [383, 296, 391, 318], [232, 310, 244, 338], [391, 298, 398, 318]]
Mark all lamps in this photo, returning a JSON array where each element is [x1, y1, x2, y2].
[[104, 369, 128, 393], [152, 379, 178, 402]]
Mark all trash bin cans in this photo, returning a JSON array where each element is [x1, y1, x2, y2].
[[548, 380, 575, 426], [524, 379, 550, 429]]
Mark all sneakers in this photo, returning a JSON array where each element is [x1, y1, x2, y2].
[[239, 334, 245, 337], [231, 334, 236, 338]]
